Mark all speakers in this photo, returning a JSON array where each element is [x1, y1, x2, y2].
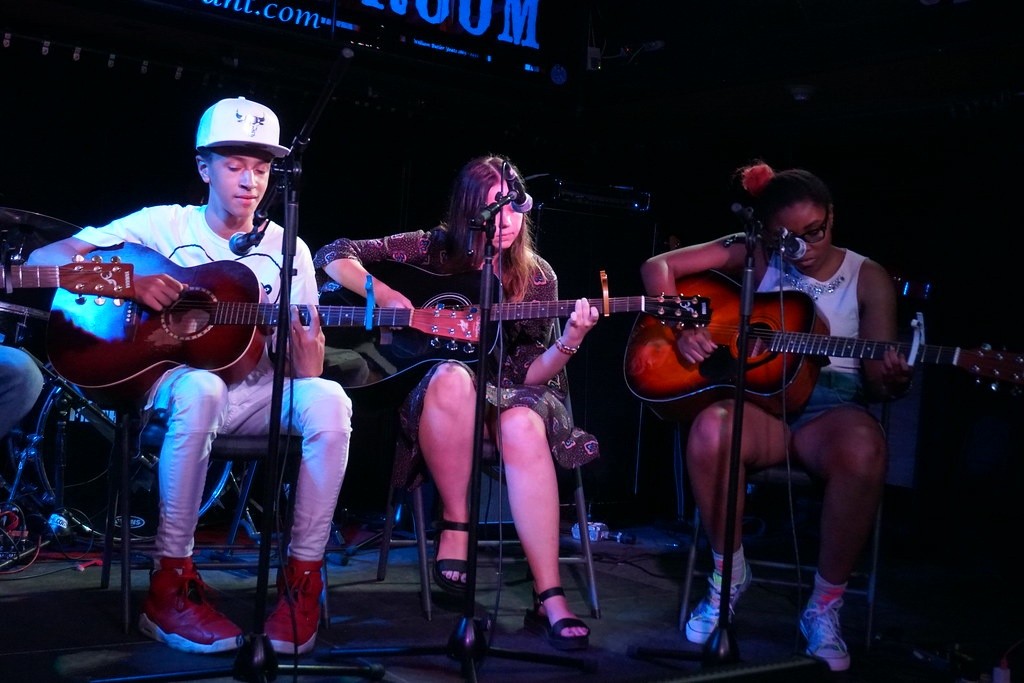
[[415, 462, 519, 541], [532, 199, 660, 509]]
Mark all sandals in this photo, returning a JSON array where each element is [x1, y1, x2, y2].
[[524, 587, 591, 651], [432, 507, 469, 597]]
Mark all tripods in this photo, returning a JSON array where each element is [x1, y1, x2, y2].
[[315, 191, 603, 683], [626, 220, 779, 668], [0, 346, 153, 571], [344, 484, 418, 556], [90, 48, 385, 683]]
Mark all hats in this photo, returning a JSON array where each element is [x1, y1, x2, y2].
[[196, 96, 291, 158]]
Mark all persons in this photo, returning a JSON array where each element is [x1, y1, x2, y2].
[[29, 95, 355, 654], [312, 152, 599, 650], [0, 344, 45, 433], [638, 163, 914, 672]]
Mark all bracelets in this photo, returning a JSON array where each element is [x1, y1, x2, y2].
[[555, 338, 580, 356]]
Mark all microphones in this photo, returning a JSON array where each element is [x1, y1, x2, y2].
[[229, 231, 264, 255], [505, 163, 533, 212], [777, 226, 806, 260]]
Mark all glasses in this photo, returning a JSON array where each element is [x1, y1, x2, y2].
[[796, 202, 829, 243]]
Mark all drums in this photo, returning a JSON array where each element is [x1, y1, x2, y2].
[[8, 348, 234, 548]]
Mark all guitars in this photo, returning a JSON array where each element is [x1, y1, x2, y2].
[[319, 257, 713, 410], [622, 269, 1024, 423], [46, 240, 481, 402]]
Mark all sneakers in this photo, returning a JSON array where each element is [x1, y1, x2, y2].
[[138, 565, 243, 654], [798, 596, 850, 672], [262, 570, 324, 654], [685, 558, 752, 644]]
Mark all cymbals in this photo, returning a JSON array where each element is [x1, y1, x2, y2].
[[0, 206, 83, 237]]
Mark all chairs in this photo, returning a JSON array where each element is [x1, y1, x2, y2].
[[374, 308, 600, 624], [101, 398, 333, 634], [678, 386, 897, 653]]
